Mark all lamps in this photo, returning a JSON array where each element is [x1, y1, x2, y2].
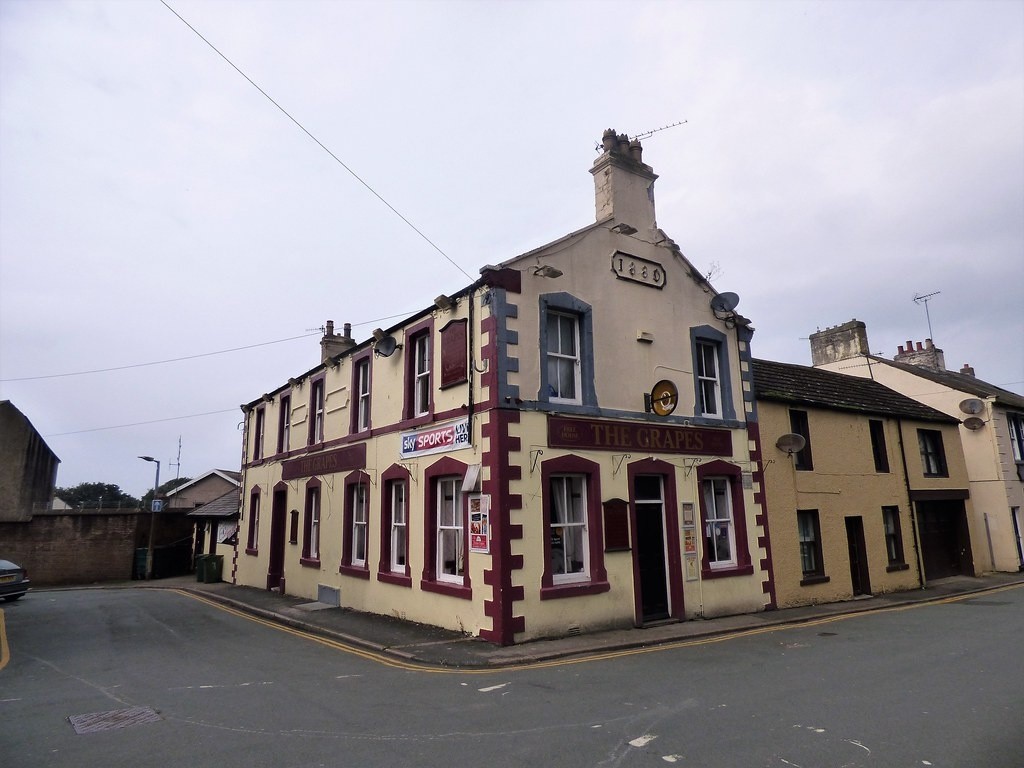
[[240, 404, 254, 414], [434, 293, 457, 311], [287, 377, 302, 387], [261, 392, 274, 402], [534, 265, 563, 279], [612, 224, 637, 236], [323, 356, 340, 369], [372, 328, 386, 341], [724, 315, 751, 329]]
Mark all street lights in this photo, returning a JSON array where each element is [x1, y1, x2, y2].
[[138, 455, 160, 577]]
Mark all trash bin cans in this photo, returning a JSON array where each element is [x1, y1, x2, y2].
[[195, 554, 225, 583]]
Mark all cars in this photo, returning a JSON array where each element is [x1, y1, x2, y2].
[[1, 558, 30, 601]]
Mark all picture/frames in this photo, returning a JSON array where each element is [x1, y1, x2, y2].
[[681, 501, 697, 528], [684, 554, 700, 582], [683, 529, 698, 555]]
